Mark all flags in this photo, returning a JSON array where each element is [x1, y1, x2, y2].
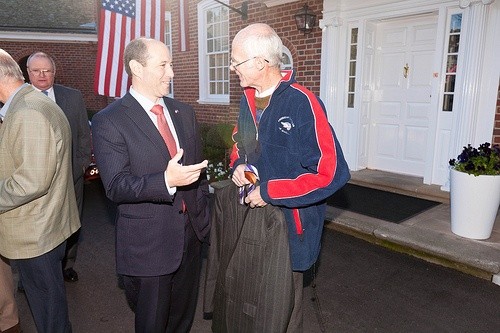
[[93, 0, 165, 99]]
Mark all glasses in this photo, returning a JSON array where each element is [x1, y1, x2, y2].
[[27, 68, 54, 76], [229, 56, 269, 70]]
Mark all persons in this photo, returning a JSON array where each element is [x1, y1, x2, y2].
[[26, 51, 92, 283], [0, 48, 83, 333], [91, 36, 215, 333], [229, 22, 350, 333]]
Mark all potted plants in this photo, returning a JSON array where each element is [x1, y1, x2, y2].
[[447, 142, 500, 240]]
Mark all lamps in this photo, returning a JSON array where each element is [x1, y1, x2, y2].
[[291, 2, 316, 37]]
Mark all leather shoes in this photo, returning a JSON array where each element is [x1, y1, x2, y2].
[[63, 268, 79, 284]]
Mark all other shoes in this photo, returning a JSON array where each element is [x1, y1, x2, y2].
[[204, 312, 213, 322]]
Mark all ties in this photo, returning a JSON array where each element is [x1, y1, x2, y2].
[[150, 104, 186, 214], [41, 89, 48, 96]]
